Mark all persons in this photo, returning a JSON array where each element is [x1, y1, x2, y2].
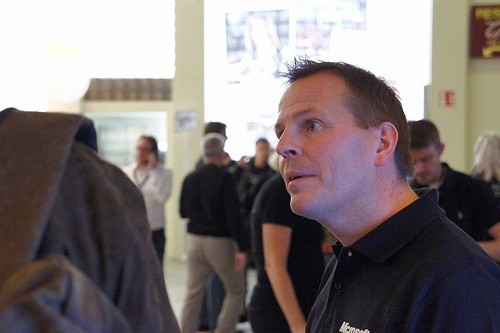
[[0, 107, 181, 333], [123, 122, 337, 333], [275, 54, 500, 333]]
